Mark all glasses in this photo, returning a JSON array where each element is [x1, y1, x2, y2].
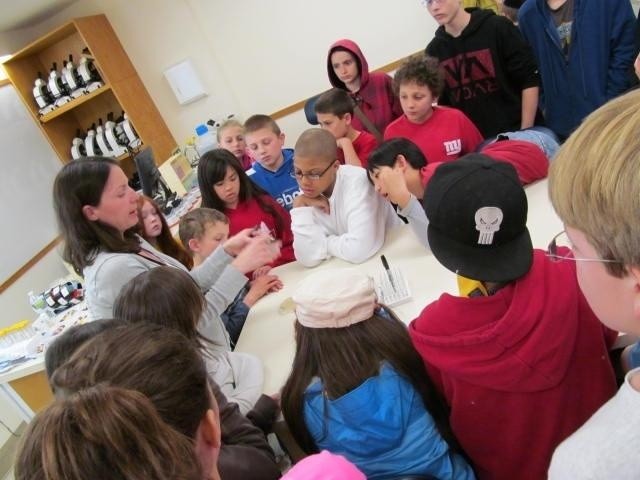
[[545, 228, 631, 264], [288, 159, 336, 180]]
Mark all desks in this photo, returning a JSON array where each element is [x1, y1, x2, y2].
[[0, 301, 95, 427], [229, 176, 631, 429]]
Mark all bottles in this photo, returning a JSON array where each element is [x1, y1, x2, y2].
[[195, 126, 220, 155], [0, 318, 35, 349]]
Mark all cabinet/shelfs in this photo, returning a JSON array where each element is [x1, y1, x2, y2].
[[0, 12, 182, 196]]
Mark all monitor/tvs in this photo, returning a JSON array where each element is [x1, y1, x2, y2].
[[129, 145, 177, 211]]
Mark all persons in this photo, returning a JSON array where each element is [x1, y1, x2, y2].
[[2, 2, 640, 479]]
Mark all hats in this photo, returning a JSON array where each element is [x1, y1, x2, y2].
[[423, 151, 534, 282], [292, 267, 378, 328]]
